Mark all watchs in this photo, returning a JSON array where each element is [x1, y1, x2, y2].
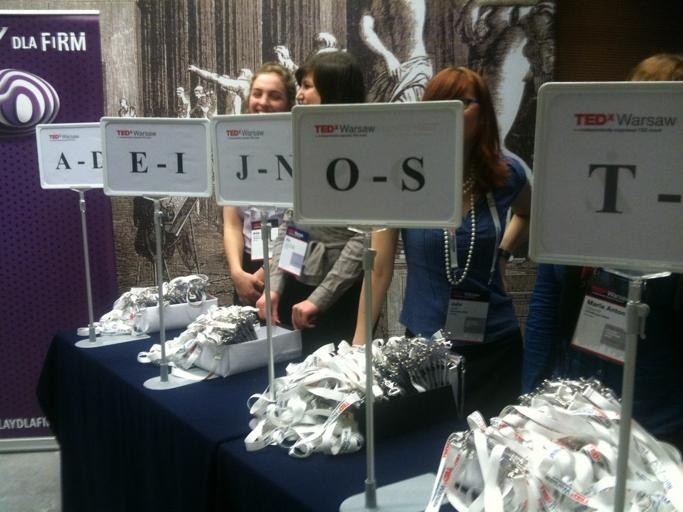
[[495, 247, 512, 261]]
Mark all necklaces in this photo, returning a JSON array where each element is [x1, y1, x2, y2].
[[442, 163, 476, 287]]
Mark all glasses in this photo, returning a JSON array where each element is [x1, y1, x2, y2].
[[457, 97, 482, 109]]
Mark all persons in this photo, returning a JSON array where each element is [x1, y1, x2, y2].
[[353, 67, 532, 410], [256, 52, 366, 355], [522, 53, 683, 457], [223, 63, 298, 308]]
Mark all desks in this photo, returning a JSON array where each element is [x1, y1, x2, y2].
[[36, 328, 467, 512]]
